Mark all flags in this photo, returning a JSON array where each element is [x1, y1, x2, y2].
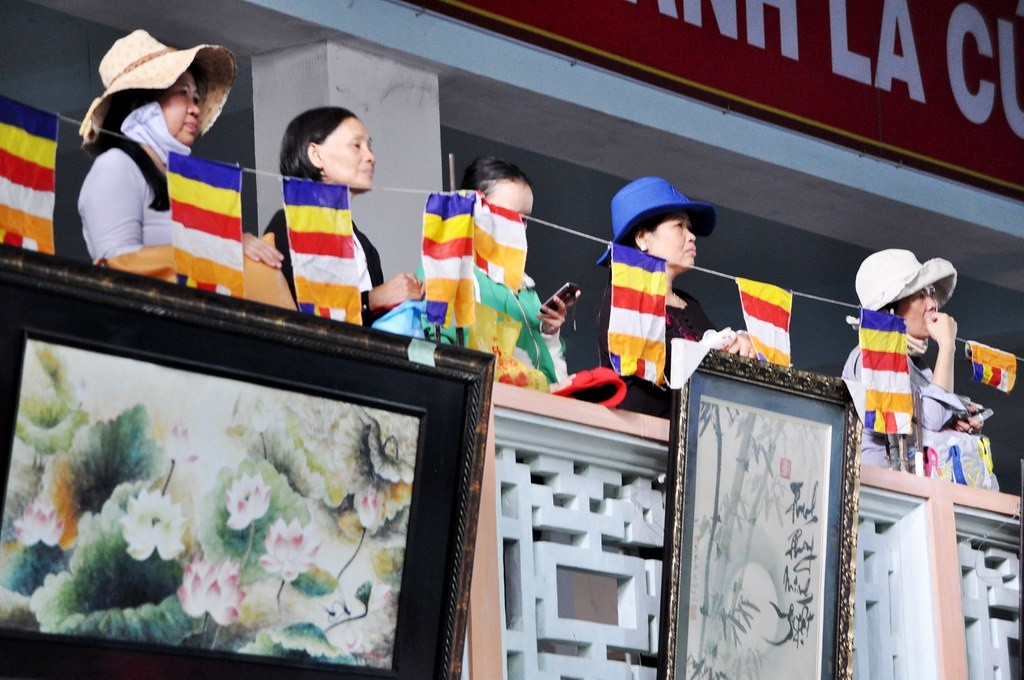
[[963, 336, 1016, 394], [734, 275, 792, 368], [420, 190, 553, 393], [597, 235, 669, 394], [856, 306, 913, 436], [166, 151, 246, 299], [281, 178, 363, 326], [1, 93, 59, 255]]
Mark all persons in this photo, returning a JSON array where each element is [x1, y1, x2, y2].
[[76, 29, 285, 273], [843, 245, 983, 434], [417, 155, 573, 390], [265, 106, 424, 327], [595, 176, 754, 417]]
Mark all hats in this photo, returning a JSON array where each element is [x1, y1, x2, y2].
[[596, 177, 717, 268], [78, 29, 238, 159], [846, 248, 958, 332]]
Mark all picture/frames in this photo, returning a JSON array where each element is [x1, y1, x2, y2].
[[0, 244, 496, 680], [655, 349, 862, 680]]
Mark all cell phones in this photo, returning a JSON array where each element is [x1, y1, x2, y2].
[[540, 282, 577, 314]]
